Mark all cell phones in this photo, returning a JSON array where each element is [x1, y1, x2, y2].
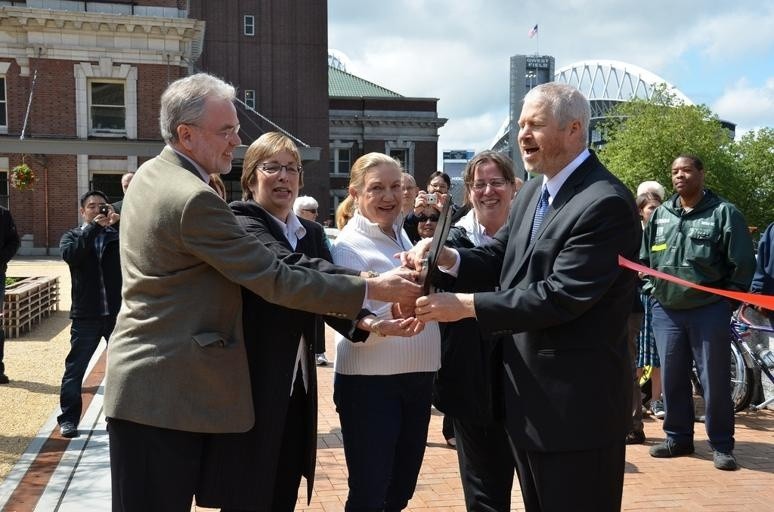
[[98, 205, 108, 217]]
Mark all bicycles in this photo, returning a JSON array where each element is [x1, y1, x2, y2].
[[691, 300, 773, 415]]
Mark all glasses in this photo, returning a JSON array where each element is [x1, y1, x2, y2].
[[468, 178, 506, 189], [419, 215, 436, 222], [304, 209, 316, 213], [182, 123, 241, 135], [258, 162, 302, 176]]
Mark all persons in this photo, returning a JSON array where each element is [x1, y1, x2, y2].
[[112, 170, 526, 451], [104, 72, 441, 512], [625, 180, 665, 445], [748, 223, 774, 330], [0, 204, 21, 385], [638, 154, 757, 470], [393, 82, 644, 512], [55, 188, 124, 439]]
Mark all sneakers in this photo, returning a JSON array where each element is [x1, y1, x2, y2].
[[626, 431, 645, 444], [315, 353, 329, 364], [712, 448, 736, 470], [61, 421, 78, 438], [0, 375, 9, 384], [651, 399, 665, 417], [650, 441, 694, 457]]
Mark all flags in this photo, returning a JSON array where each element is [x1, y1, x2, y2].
[[528, 24, 538, 39]]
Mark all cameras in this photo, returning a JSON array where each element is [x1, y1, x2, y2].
[[424, 194, 437, 205]]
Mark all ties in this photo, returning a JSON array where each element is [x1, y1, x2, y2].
[[530, 185, 550, 244]]
